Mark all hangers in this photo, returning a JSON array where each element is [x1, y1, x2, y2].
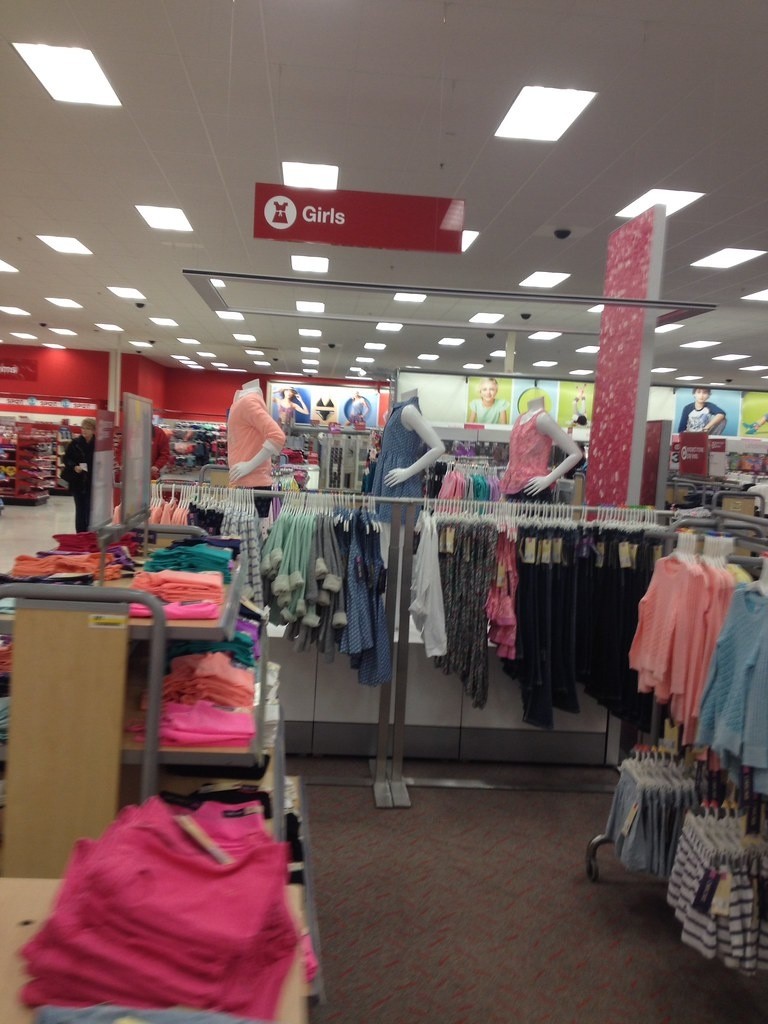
[[422, 498, 667, 535], [276, 490, 383, 535], [684, 801, 767, 860], [150, 482, 255, 517], [617, 746, 695, 794], [744, 557, 768, 598], [268, 468, 297, 490], [663, 532, 734, 569], [446, 457, 506, 476]]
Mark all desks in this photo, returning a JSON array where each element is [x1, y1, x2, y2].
[[1, 878, 310, 1024]]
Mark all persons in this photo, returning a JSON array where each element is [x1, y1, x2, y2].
[[344, 391, 370, 427], [62, 418, 95, 533], [115, 422, 170, 478], [227, 379, 286, 526], [678, 386, 727, 437], [742, 412, 768, 435], [469, 378, 508, 424], [371, 389, 445, 569], [498, 396, 583, 517], [272, 386, 310, 425], [571, 384, 588, 426]]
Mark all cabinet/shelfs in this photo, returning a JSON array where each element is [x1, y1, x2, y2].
[[0, 421, 122, 506], [0, 524, 325, 1010]]
